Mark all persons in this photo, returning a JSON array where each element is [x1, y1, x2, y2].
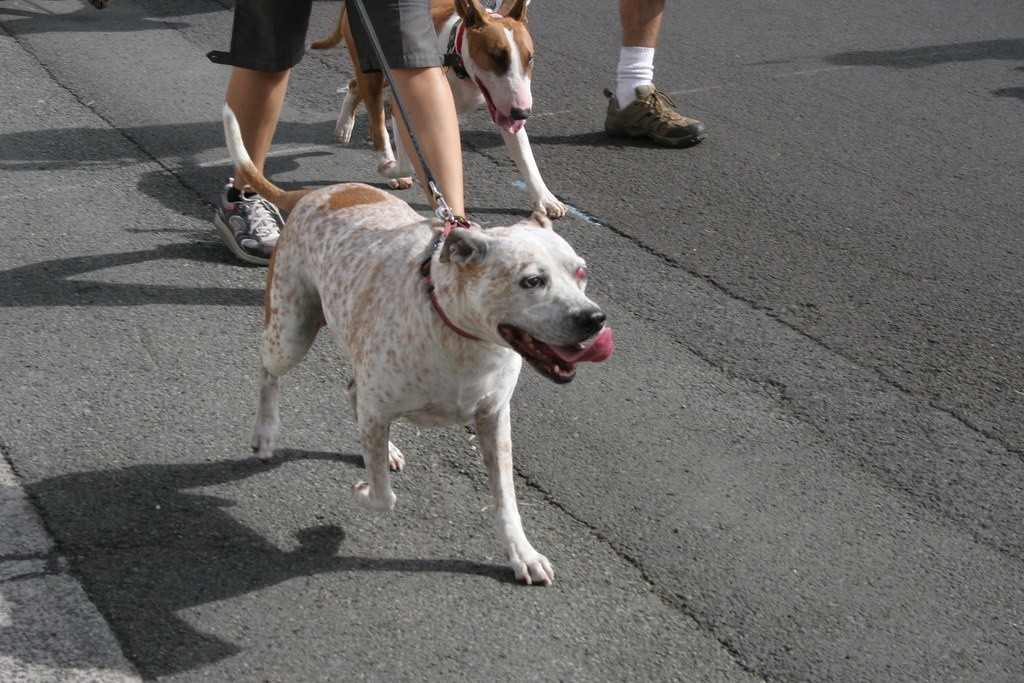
[[205, 0, 466, 266], [489, 0, 709, 146]]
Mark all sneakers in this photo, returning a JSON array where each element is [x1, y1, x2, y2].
[[603, 84, 708, 145], [214, 176, 286, 264]]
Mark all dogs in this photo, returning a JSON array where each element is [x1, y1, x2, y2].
[[304, 0, 571, 225], [221, 99, 615, 587]]
